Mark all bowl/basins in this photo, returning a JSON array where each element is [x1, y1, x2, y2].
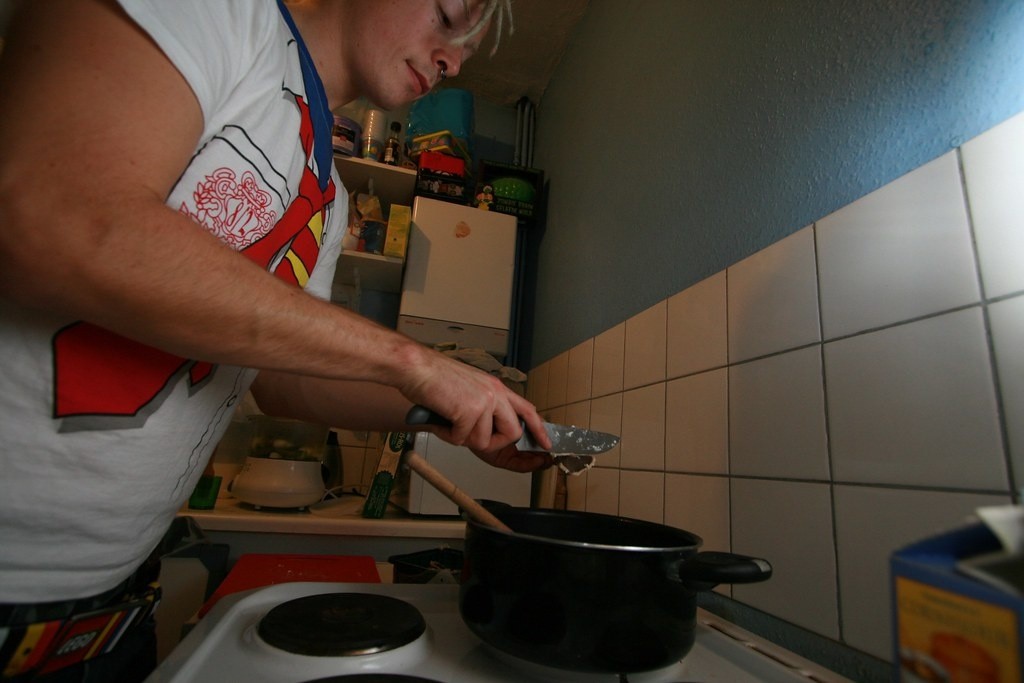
[[386, 547, 464, 584]]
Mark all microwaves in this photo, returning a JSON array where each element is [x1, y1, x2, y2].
[[388, 431, 532, 515]]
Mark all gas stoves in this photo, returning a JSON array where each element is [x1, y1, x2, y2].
[[142, 582, 860, 683]]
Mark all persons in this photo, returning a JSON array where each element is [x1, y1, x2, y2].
[[0, 0, 552, 683]]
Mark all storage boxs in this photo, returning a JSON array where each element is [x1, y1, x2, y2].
[[383, 204, 412, 262]]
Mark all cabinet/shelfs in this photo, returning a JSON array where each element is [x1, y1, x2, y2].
[[333, 152, 417, 295]]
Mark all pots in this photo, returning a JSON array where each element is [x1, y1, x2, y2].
[[457, 499, 772, 671], [231, 457, 326, 511]]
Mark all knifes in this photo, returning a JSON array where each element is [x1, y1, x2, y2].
[[406, 404, 622, 453]]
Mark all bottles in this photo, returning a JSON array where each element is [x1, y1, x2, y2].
[[381, 121, 402, 166]]
